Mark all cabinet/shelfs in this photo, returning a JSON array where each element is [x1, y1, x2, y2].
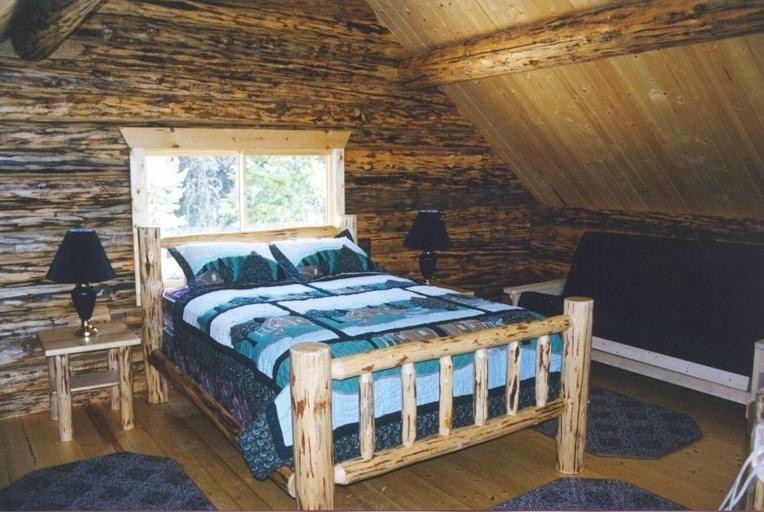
[[41, 319, 142, 440]]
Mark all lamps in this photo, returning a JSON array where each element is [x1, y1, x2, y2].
[[47, 227, 113, 337], [404, 206, 452, 285]]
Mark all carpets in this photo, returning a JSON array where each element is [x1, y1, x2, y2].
[[0, 451, 217, 512], [486, 385, 703, 512]]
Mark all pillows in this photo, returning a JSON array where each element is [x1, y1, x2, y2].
[[169, 237, 378, 291]]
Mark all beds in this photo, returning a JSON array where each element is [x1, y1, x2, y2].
[[137, 215, 595, 512]]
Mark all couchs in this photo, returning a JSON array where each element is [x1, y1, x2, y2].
[[505, 231, 764, 417]]
